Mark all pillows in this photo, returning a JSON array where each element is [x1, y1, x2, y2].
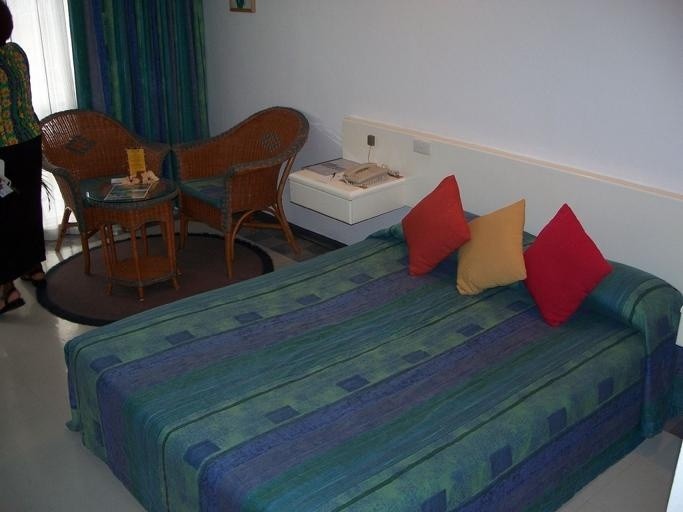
[[520, 203, 611, 328], [398, 174, 473, 279], [452, 198, 528, 297]]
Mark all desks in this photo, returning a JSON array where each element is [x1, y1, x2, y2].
[[74, 173, 183, 303]]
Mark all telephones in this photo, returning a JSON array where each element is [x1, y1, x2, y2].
[[341, 163, 385, 185]]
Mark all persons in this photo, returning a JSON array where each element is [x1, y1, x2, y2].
[[0, 1, 46, 316]]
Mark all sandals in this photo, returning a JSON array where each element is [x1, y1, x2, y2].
[[21, 270, 46, 286], [0, 286, 26, 313]]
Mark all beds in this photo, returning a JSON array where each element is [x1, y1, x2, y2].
[[61, 207, 683, 511]]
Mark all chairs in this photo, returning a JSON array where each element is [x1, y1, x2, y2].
[[169, 103, 310, 283], [35, 107, 174, 277]]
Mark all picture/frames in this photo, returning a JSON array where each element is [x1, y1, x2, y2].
[[228, 1, 255, 13]]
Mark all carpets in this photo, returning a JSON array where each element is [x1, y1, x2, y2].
[[35, 231, 275, 329]]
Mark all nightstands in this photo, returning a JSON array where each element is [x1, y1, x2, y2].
[[285, 167, 404, 225]]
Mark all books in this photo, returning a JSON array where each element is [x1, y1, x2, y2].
[[300, 157, 362, 182], [103, 183, 152, 201]]
